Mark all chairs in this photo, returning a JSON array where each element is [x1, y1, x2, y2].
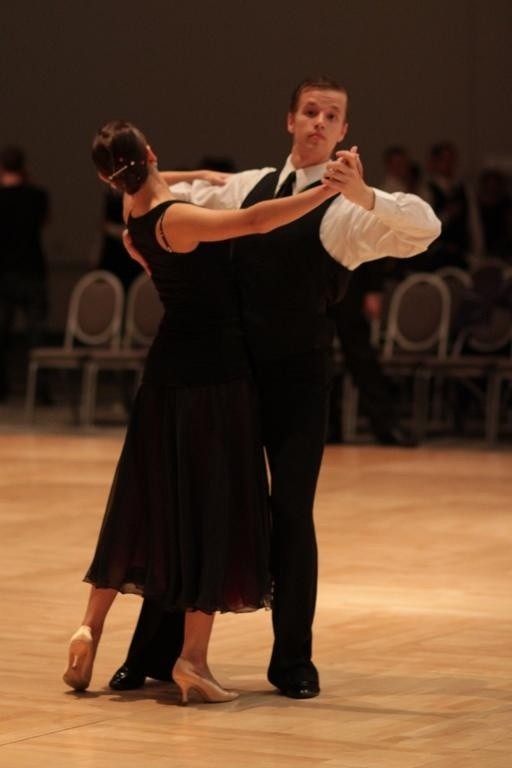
[[78, 271, 165, 426], [25, 270, 125, 424], [332, 267, 512, 443]]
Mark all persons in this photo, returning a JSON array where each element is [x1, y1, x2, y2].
[[63, 77, 441, 703], [1, 143, 234, 406], [330, 141, 510, 446]]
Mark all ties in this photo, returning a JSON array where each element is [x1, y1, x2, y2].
[[274, 173, 296, 200]]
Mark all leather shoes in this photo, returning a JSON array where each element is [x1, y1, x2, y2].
[[108, 662, 147, 690], [267, 662, 320, 698], [375, 429, 418, 447]]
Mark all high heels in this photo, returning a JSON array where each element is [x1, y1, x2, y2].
[[63, 628, 93, 690], [172, 659, 241, 705]]
